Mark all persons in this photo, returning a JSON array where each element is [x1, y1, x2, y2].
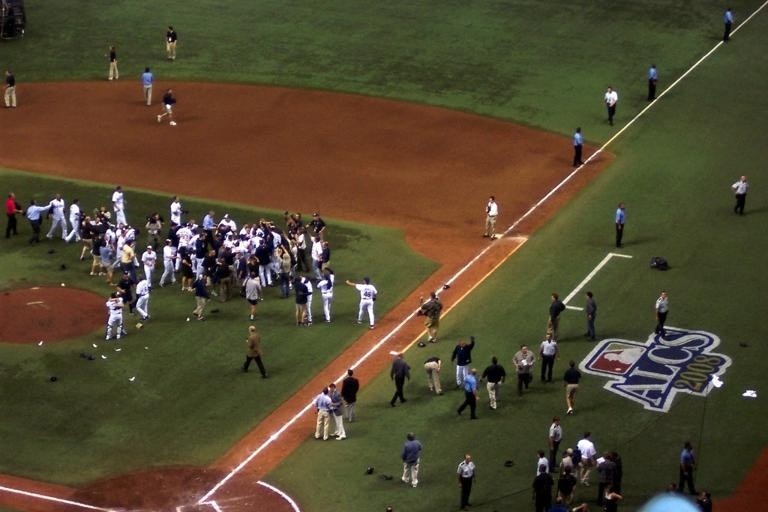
[[7, 185, 336, 340], [166, 26, 177, 60], [157, 88, 177, 125], [2, 70, 17, 108], [543, 292, 596, 341], [142, 67, 154, 105], [732, 176, 749, 216], [573, 127, 584, 167], [654, 291, 669, 334], [563, 360, 581, 414], [401, 433, 423, 487], [421, 292, 442, 343], [666, 440, 711, 511], [483, 196, 499, 240], [616, 202, 626, 248], [239, 325, 268, 379], [104, 45, 119, 80], [724, 8, 734, 41], [647, 64, 658, 102], [532, 416, 624, 512], [345, 277, 377, 329], [456, 454, 475, 510], [311, 334, 561, 440], [604, 87, 618, 126]]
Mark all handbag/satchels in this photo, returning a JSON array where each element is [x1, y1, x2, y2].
[[650, 256, 668, 270], [240, 286, 246, 297]]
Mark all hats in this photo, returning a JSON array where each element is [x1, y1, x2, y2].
[[223, 213, 230, 218], [146, 245, 152, 248], [166, 238, 173, 243], [312, 212, 318, 216], [365, 277, 370, 283]]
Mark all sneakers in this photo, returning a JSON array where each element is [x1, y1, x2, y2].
[[108, 78, 113, 80], [315, 434, 320, 439], [297, 323, 301, 326], [489, 406, 497, 411], [307, 323, 312, 326], [198, 317, 206, 321], [411, 483, 418, 487], [491, 234, 496, 238], [567, 407, 573, 414], [325, 320, 331, 323], [169, 122, 176, 125], [157, 115, 161, 122], [261, 374, 267, 378], [99, 272, 106, 276], [80, 256, 85, 261], [661, 331, 666, 334], [168, 56, 171, 59], [584, 333, 590, 336], [553, 464, 559, 467], [46, 233, 54, 239], [655, 331, 659, 334], [470, 416, 478, 419], [251, 315, 255, 320], [146, 315, 151, 319], [172, 56, 176, 59], [140, 317, 145, 320], [115, 77, 119, 79], [171, 281, 178, 284], [368, 325, 375, 329], [583, 481, 590, 486], [12, 105, 16, 107], [90, 271, 97, 276], [5, 105, 10, 107], [358, 320, 362, 324], [329, 433, 338, 436], [457, 409, 462, 415], [302, 322, 307, 326], [182, 287, 188, 292], [390, 401, 396, 407], [401, 399, 407, 402], [241, 368, 247, 372], [105, 337, 111, 340], [431, 338, 437, 343], [193, 311, 198, 316], [402, 478, 409, 483], [158, 282, 165, 288], [550, 469, 558, 473], [429, 387, 434, 391], [461, 506, 467, 510], [466, 502, 471, 506], [483, 232, 488, 236], [323, 436, 328, 440], [335, 436, 347, 440], [189, 287, 194, 293], [437, 391, 444, 396], [588, 338, 595, 341], [457, 385, 462, 389], [427, 336, 432, 342], [116, 335, 121, 340]]
